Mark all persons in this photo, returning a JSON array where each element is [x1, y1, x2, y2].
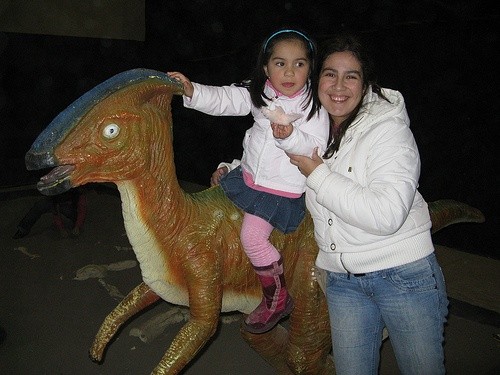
[[168, 28, 328, 336], [10, 184, 89, 244], [209, 35, 448, 375]]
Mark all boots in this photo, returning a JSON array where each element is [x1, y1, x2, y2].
[[242, 254, 295, 334]]
